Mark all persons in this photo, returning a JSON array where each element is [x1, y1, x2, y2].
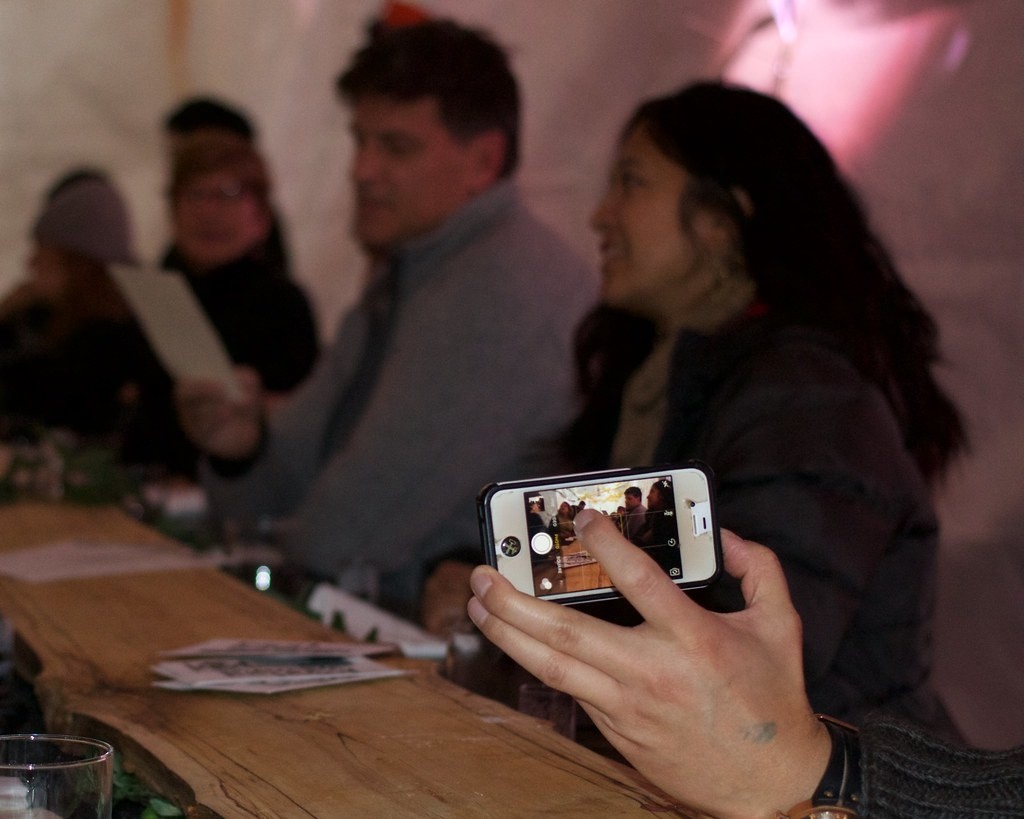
[[525, 479, 682, 581], [468, 526, 1024, 819], [178, 14, 598, 622], [0, 97, 324, 488], [427, 80, 973, 766]]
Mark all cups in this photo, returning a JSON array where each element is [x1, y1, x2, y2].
[[0, 736, 113, 819]]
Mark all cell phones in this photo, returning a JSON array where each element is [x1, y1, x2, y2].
[[476, 458, 723, 605]]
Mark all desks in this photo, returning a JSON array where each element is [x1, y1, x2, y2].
[[534, 538, 614, 595], [0, 505, 710, 819]]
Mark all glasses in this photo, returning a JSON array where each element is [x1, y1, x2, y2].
[[178, 182, 249, 205]]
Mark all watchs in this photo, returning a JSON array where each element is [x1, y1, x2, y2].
[[775, 714, 863, 819]]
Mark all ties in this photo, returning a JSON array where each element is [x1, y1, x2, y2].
[[312, 258, 403, 460]]
